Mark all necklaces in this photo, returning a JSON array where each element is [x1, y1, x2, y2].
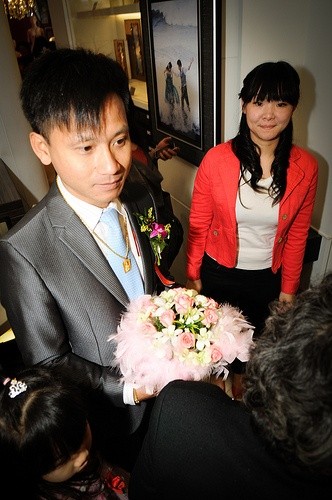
[[91, 214, 133, 274]]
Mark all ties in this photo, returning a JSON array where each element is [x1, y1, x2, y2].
[[98, 208, 146, 302]]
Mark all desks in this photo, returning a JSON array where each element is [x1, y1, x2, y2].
[[0, 158, 26, 337]]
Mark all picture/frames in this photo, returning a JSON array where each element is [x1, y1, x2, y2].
[[138, 0, 223, 167]]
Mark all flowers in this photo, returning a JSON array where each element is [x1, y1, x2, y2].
[[136, 207, 171, 266], [108, 287, 257, 396]]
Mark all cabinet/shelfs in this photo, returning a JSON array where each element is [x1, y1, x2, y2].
[[47, 0, 149, 112]]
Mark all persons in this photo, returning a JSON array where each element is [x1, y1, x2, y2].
[[0, 48, 179, 475], [27, 15, 45, 54], [128, 273, 332, 500], [185, 61, 320, 403], [1, 368, 129, 500], [12, 35, 56, 79], [163, 60, 179, 112], [127, 98, 184, 286], [173, 59, 194, 112]]
